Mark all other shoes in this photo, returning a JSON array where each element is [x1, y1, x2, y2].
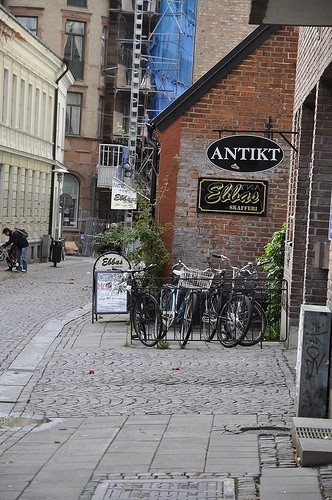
[[12, 270, 18, 271], [22, 270, 27, 271]]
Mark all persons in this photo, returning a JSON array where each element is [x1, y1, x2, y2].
[[2, 228, 29, 272]]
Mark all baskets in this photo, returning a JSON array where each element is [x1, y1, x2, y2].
[[180, 267, 214, 288]]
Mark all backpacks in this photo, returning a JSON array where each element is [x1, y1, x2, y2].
[[12, 229, 28, 239]]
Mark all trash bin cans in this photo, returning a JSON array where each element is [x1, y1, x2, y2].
[[48, 237, 63, 262]]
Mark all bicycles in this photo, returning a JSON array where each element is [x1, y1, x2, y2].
[[200, 252, 255, 348], [0, 241, 19, 269], [222, 259, 272, 347], [112, 262, 163, 347], [160, 259, 215, 349]]
